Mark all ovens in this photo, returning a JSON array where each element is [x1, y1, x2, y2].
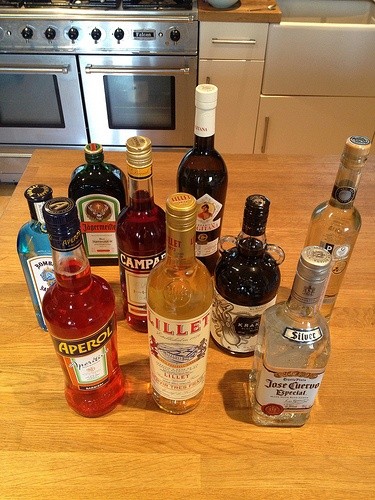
[[0, 53, 198, 183]]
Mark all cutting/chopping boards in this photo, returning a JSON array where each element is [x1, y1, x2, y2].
[[197, 0, 282, 24]]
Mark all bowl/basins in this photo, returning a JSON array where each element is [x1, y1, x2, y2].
[[208, 0, 238, 9]]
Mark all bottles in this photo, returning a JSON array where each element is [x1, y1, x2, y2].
[[247, 246, 333, 428], [115, 136, 167, 334], [177, 83, 228, 277], [145, 192, 214, 415], [303, 135, 371, 324], [17, 184, 56, 331], [42, 197, 127, 419], [68, 143, 127, 267], [210, 194, 285, 357]]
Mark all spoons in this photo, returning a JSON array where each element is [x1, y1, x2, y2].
[[248, 3, 277, 11]]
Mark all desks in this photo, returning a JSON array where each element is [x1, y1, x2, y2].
[[0, 149, 375, 500]]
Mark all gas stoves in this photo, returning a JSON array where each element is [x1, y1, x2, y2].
[[0, 0, 199, 56]]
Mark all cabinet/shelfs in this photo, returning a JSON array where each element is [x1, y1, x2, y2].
[[199, 20, 374, 158]]
